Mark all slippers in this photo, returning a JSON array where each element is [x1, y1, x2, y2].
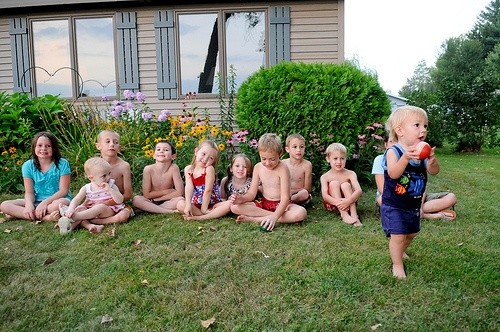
[[59, 203, 75, 222], [58, 217, 71, 234], [441, 210, 456, 221]]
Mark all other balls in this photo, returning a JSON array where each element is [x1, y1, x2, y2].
[[412, 141, 431, 160]]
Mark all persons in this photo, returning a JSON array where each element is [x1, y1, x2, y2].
[[133, 140, 185, 214], [258, 133, 312, 208], [230, 133, 307, 232], [380, 105, 440, 279], [220, 153, 252, 202], [81, 130, 135, 234], [58, 156, 124, 235], [177, 140, 230, 220], [0, 132, 72, 222], [371, 132, 456, 222], [320, 143, 362, 227]]
